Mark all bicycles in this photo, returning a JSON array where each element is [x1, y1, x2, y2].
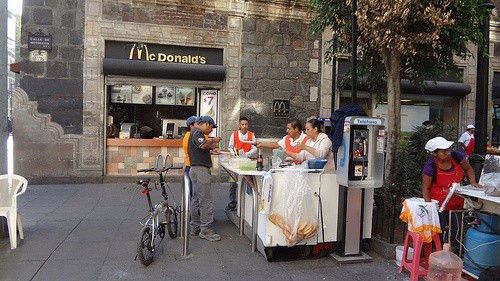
[[133, 162, 184, 265]]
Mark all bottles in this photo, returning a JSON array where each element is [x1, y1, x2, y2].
[[167, 131, 172, 139], [257, 154, 263, 171], [428, 243, 463, 281]]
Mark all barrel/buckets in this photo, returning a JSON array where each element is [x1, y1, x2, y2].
[[395, 246, 413, 266]]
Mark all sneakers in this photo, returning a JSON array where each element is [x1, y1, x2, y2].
[[199, 231, 221, 241], [191, 230, 200, 236]]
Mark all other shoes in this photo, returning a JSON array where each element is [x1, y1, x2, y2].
[[224, 206, 235, 211]]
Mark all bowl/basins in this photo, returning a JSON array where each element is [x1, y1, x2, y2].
[[231, 158, 256, 171], [308, 159, 328, 173]]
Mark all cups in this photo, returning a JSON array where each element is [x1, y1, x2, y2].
[[263, 159, 269, 170], [136, 133, 140, 139], [295, 141, 300, 149], [239, 150, 244, 158], [182, 133, 185, 140]]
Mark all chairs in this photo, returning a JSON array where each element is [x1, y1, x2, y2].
[[0, 174, 28, 249]]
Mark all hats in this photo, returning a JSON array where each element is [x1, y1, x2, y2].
[[425, 136, 454, 151], [466, 125, 475, 129], [187, 116, 199, 127], [198, 116, 218, 129], [423, 121, 432, 126]]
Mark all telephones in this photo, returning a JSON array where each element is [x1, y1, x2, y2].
[[348, 129, 370, 180]]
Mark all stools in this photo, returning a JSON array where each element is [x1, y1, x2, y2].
[[398, 232, 442, 281]]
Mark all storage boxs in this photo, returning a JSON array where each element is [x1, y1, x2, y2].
[[482, 175, 500, 198]]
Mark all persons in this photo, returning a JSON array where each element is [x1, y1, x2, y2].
[[408, 120, 436, 157], [7, 116, 12, 138], [279, 118, 335, 174], [182, 115, 223, 241], [224, 117, 257, 211], [458, 124, 476, 160], [138, 125, 153, 138], [255, 118, 311, 164], [421, 137, 483, 251]]
